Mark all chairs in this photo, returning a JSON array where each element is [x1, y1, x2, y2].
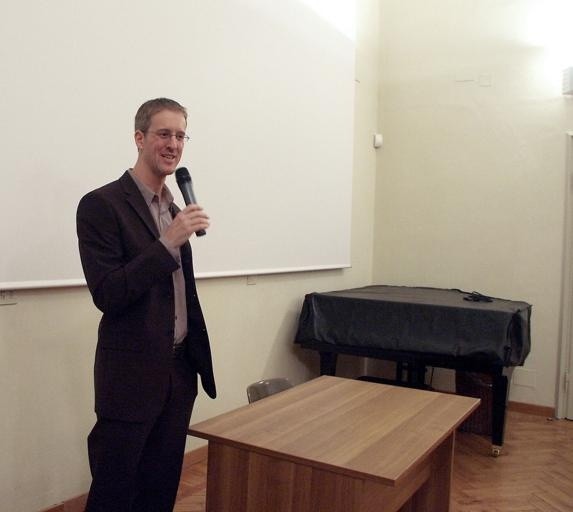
[[246, 377, 293, 404]]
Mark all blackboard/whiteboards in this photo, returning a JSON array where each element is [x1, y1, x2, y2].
[[0, 1, 356, 291]]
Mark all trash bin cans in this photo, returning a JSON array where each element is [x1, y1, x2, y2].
[[455, 370, 493, 434]]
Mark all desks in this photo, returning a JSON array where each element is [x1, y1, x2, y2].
[[187, 375, 482, 512], [293, 284, 531, 457]]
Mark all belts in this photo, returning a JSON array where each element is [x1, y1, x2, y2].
[[170, 344, 190, 361]]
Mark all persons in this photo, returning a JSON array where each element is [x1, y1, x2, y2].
[[77, 99, 216, 512]]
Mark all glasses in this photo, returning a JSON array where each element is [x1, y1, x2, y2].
[[144, 130, 190, 144]]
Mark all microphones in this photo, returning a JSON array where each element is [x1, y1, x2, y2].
[[174, 164, 208, 240]]
